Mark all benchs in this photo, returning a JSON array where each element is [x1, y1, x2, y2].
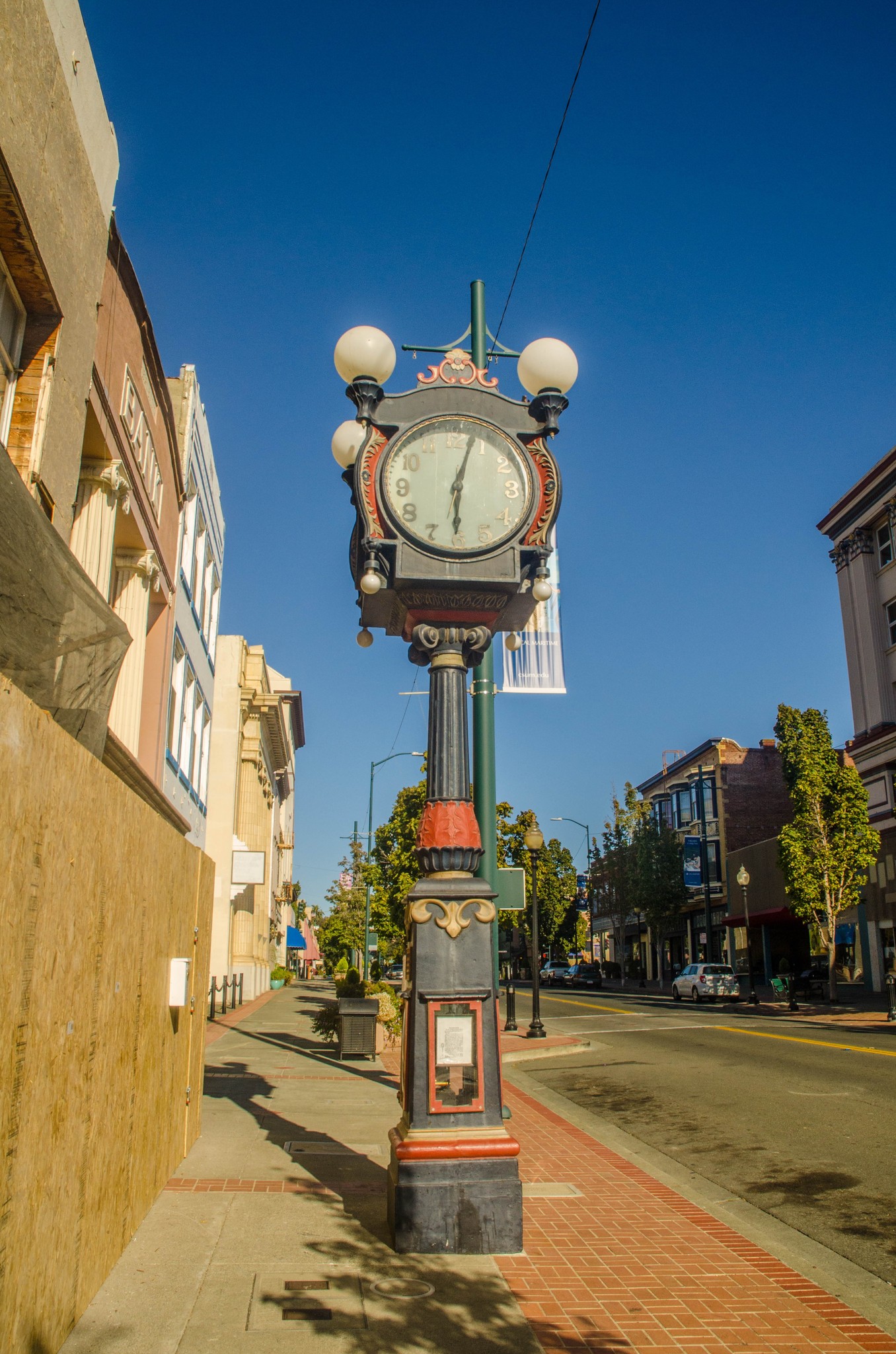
[[789, 975, 825, 1005]]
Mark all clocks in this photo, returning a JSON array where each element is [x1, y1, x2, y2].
[[384, 412, 539, 557]]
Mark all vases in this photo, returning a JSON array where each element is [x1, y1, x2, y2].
[[373, 1021, 386, 1056]]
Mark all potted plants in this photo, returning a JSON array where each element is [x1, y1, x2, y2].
[[333, 957, 349, 983], [278, 968, 286, 988], [270, 969, 282, 990]]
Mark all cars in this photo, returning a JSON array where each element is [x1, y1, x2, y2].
[[386, 970, 390, 977], [672, 963, 740, 1004], [562, 965, 602, 989]]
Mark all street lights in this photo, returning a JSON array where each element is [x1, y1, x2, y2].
[[550, 817, 595, 965], [331, 325, 580, 1258], [363, 751, 426, 983], [736, 862, 760, 1004], [634, 904, 646, 987], [524, 811, 547, 1039]]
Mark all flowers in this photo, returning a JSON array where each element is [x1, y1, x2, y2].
[[310, 978, 402, 1051]]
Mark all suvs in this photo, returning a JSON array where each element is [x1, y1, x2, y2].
[[389, 965, 403, 979]]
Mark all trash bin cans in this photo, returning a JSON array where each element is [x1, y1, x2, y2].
[[337, 996, 380, 1062]]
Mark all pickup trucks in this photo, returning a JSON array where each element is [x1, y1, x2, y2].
[[539, 961, 571, 986]]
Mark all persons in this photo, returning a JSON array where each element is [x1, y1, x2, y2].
[[687, 855, 701, 868], [577, 885, 588, 900]]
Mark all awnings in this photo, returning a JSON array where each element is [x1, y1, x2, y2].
[[287, 925, 307, 951]]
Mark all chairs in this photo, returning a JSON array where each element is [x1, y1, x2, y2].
[[768, 978, 792, 1003], [784, 977, 791, 1000]]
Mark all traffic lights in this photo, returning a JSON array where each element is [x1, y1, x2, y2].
[[543, 953, 548, 958], [298, 902, 303, 914]]
